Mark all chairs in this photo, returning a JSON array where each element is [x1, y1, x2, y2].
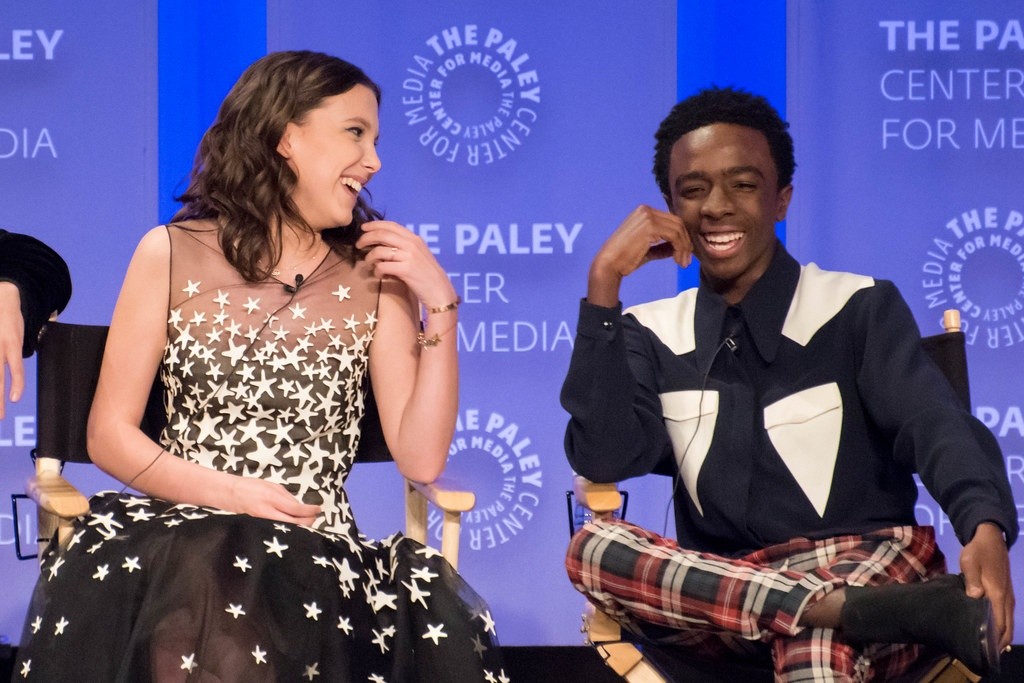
[[24, 308, 478, 573], [566, 309, 984, 683]]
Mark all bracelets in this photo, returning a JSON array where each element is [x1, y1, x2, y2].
[[418, 322, 458, 352], [425, 295, 462, 313]]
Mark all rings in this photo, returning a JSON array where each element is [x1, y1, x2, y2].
[[1004, 645, 1011, 652], [391, 247, 397, 261]]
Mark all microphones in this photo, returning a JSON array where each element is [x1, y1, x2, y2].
[[730, 321, 745, 338], [295, 274, 303, 286]]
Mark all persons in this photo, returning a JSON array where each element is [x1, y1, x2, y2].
[[559, 90, 1020, 683], [11, 49, 511, 683], [0, 228, 72, 421]]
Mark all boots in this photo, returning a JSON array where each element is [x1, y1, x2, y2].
[[839, 572, 1001, 679]]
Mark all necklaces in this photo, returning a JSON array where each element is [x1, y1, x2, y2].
[[271, 243, 322, 276]]
